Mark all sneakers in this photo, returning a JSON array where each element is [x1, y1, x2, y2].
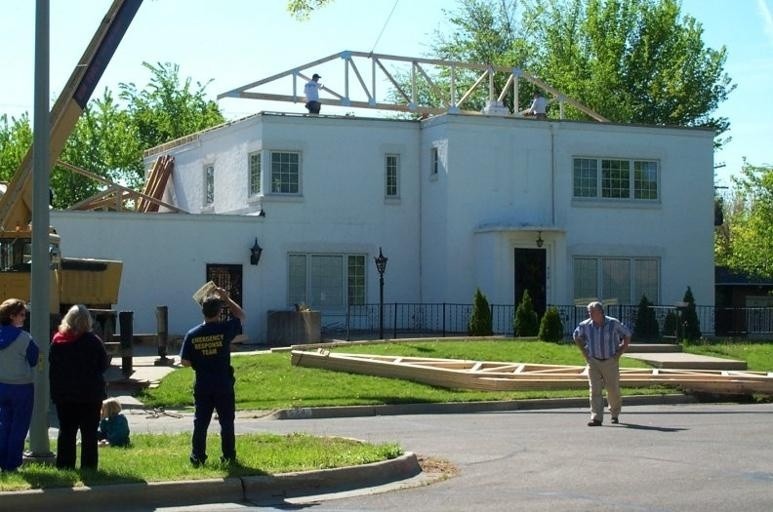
[[588, 419, 601, 425], [612, 417, 618, 423]]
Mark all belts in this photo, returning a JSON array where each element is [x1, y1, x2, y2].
[[592, 356, 608, 361]]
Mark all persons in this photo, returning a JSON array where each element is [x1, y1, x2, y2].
[[180, 287, 245, 467], [96, 401, 130, 450], [528, 90, 552, 118], [49, 305, 111, 473], [305, 75, 322, 112], [573, 301, 632, 426], [1, 298, 39, 473]]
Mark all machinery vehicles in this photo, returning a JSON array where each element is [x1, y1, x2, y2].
[[0, 0, 143, 373]]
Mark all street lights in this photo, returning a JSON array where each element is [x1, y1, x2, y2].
[[373, 247, 388, 339]]
